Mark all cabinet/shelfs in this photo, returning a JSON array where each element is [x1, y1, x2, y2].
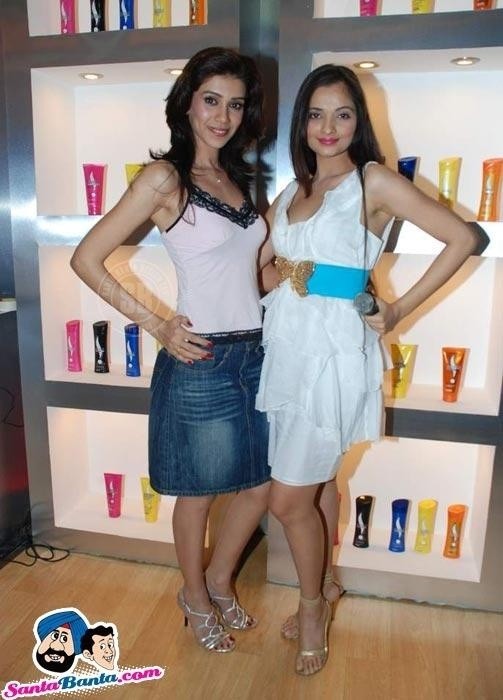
[[0, 0, 503, 616]]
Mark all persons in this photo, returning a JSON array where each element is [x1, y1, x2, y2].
[[254, 65, 476, 677], [69, 48, 285, 654]]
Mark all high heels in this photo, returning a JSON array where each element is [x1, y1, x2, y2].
[[281, 574, 346, 639], [177, 587, 236, 653], [207, 589, 257, 629], [295, 593, 332, 676]]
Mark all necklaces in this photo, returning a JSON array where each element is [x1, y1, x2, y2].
[[177, 348, 180, 353], [216, 176, 223, 184]]
